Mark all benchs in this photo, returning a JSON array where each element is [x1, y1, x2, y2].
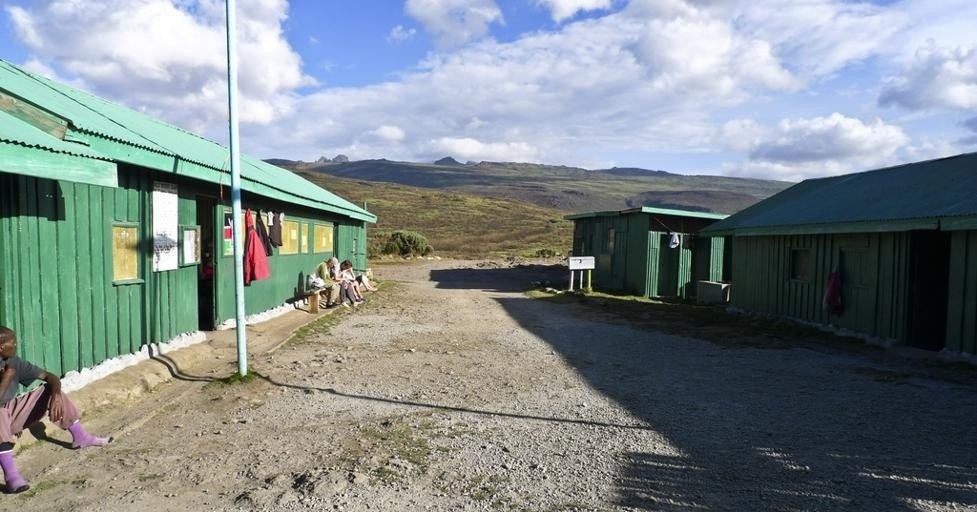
[[304, 282, 340, 314]]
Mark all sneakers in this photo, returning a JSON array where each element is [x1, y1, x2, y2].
[[326, 287, 377, 308]]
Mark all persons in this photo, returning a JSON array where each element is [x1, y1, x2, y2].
[[0, 325, 113, 493], [330, 258, 378, 307], [308, 257, 342, 309]]
